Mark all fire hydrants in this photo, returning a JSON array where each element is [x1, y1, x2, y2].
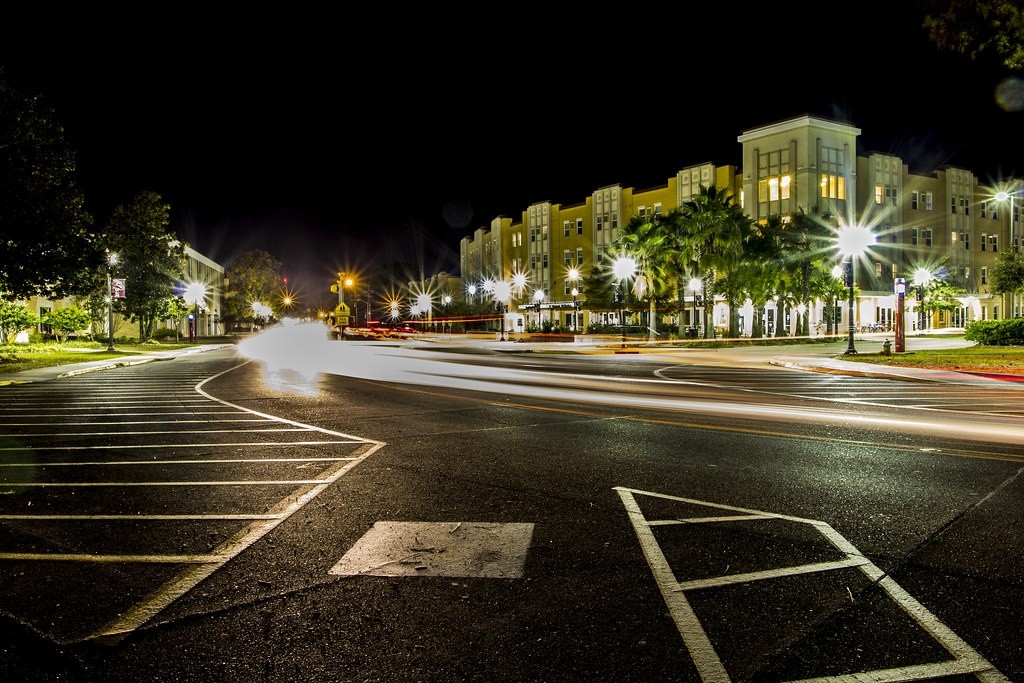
[[882, 338, 892, 354]]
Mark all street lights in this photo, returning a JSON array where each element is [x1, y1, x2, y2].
[[995, 192, 1014, 249], [535, 291, 544, 330], [839, 226, 863, 353], [915, 269, 931, 331], [569, 270, 579, 332], [184, 283, 206, 345], [106, 251, 117, 351], [613, 258, 635, 348], [689, 278, 701, 330]]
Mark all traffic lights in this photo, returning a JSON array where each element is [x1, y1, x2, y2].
[[330, 283, 339, 294], [343, 276, 354, 289]]
